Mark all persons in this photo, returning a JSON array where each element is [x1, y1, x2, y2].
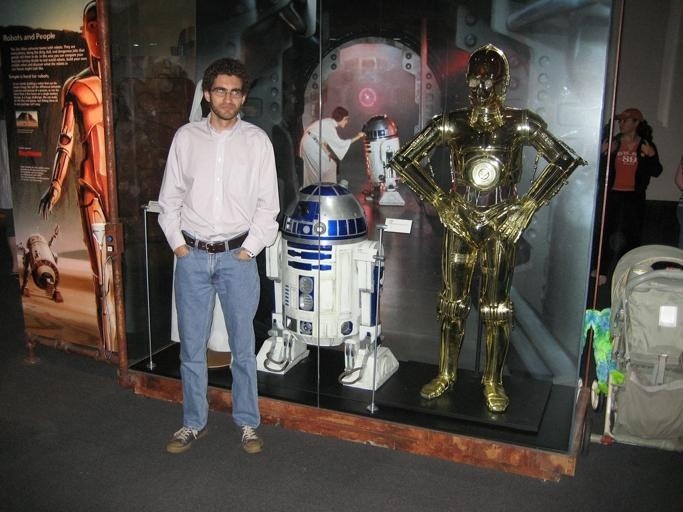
[[672, 152, 683, 249], [386, 41, 585, 416], [37, 1, 122, 366], [592, 105, 663, 285], [156, 54, 281, 456], [298, 105, 367, 190], [589, 124, 609, 279]]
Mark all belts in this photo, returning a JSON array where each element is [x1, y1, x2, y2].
[[182, 232, 248, 252]]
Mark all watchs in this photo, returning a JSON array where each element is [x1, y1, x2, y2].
[[241, 248, 255, 259]]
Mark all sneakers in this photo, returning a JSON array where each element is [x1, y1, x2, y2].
[[239, 425, 264, 453], [166, 426, 208, 453]]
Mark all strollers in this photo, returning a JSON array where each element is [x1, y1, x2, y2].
[[580, 244, 683, 459]]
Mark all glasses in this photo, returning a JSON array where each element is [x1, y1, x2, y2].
[[214, 89, 242, 96]]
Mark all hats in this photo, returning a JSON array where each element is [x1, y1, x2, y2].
[[614, 108, 643, 120]]
